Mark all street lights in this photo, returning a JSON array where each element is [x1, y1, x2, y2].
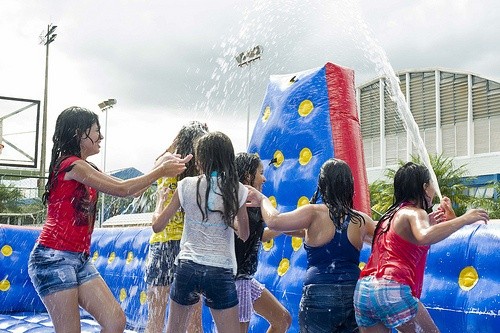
[[235, 46, 265, 147], [37, 24, 58, 198], [98, 98, 117, 227]]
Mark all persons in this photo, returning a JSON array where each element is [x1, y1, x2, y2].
[[438, 197, 456, 220], [152, 131, 250, 333], [28, 106, 193, 333], [353, 162, 490, 333], [145, 121, 209, 333], [244, 158, 376, 333], [235, 152, 292, 333]]
